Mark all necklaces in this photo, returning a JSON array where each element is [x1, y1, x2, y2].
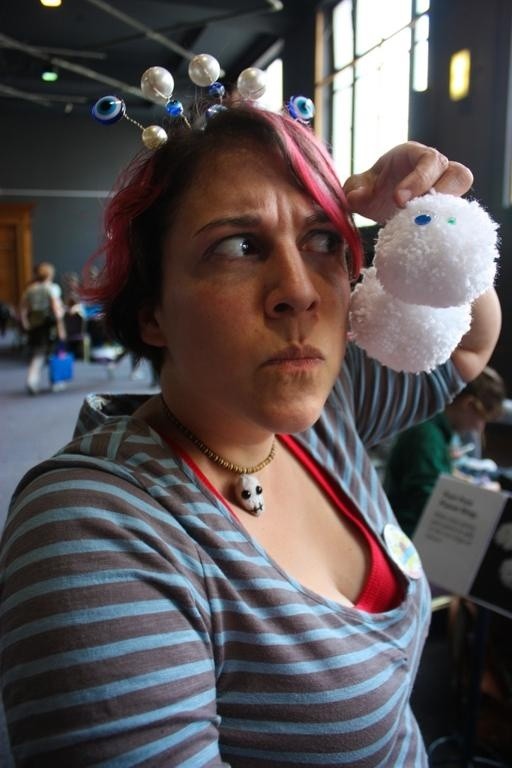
[[157, 391, 279, 518]]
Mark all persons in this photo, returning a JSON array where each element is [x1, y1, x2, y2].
[[1, 106, 502, 767], [383, 367, 505, 539], [21, 262, 68, 394]]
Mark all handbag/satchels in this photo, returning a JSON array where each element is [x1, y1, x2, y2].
[[49, 353, 74, 384]]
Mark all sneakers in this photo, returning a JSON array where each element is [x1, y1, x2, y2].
[[25, 380, 68, 397], [105, 363, 147, 381]]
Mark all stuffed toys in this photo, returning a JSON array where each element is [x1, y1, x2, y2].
[[349, 193, 502, 377]]
